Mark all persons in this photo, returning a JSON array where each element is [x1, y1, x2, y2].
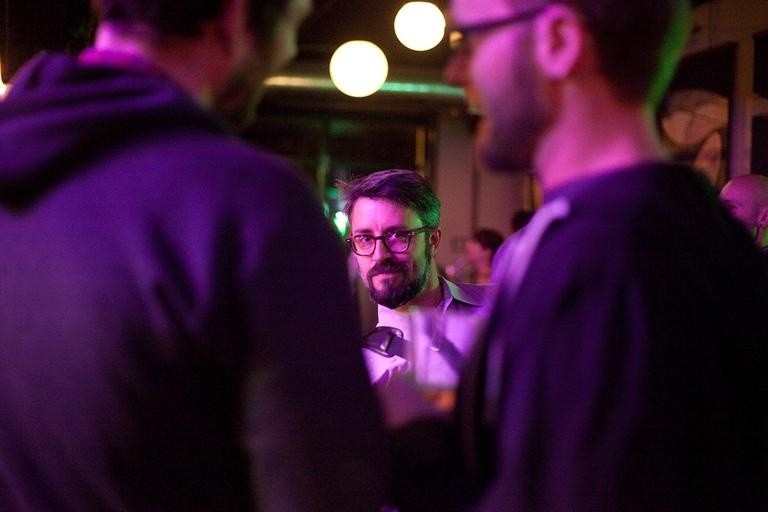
[[444, 0, 768, 512], [1, 2, 390, 507], [715, 171, 768, 249], [463, 228, 505, 286], [337, 174, 499, 426]]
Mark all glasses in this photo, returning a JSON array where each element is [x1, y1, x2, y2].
[[345, 226, 426, 257], [445, 8, 534, 60]]
